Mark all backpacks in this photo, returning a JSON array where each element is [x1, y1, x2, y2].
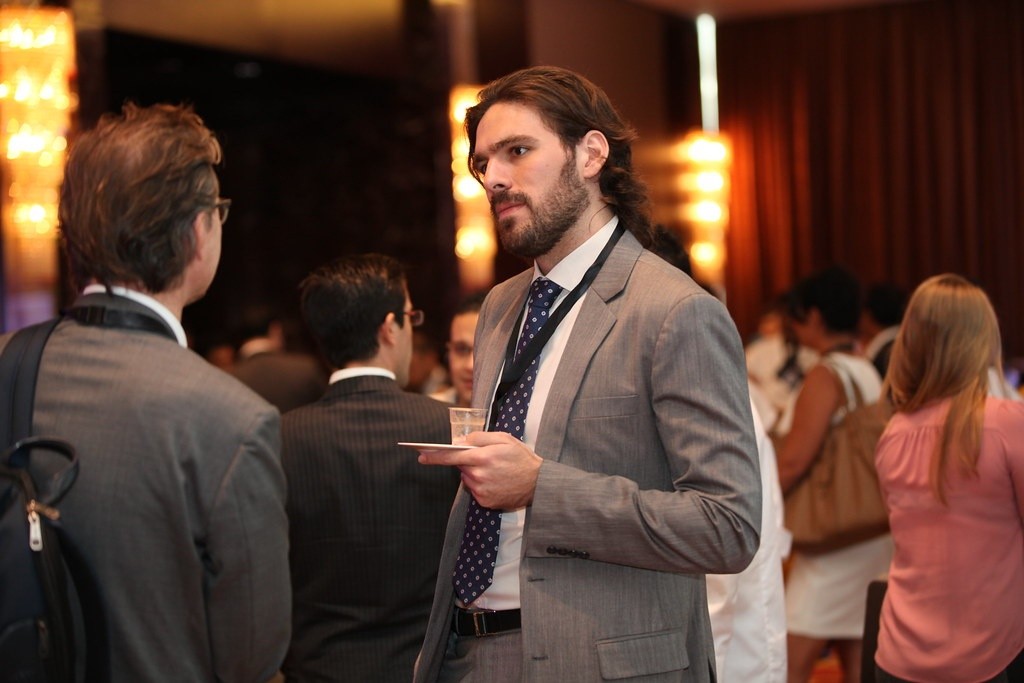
[[0, 318, 119, 683]]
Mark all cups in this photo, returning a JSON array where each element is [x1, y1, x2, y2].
[[448, 406, 488, 444]]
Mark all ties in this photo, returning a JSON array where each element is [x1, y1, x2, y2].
[[451, 278, 561, 609]]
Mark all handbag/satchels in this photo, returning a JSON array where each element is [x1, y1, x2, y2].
[[765, 360, 893, 553]]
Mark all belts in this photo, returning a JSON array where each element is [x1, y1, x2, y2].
[[446, 608, 521, 637]]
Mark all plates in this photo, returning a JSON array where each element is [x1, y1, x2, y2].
[[397, 441, 484, 455]]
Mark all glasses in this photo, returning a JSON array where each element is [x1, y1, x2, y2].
[[211, 199, 231, 224], [402, 310, 424, 327]]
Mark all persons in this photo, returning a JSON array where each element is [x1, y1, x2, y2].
[[2, 101, 295, 683], [193, 250, 486, 683], [413, 66, 763, 683], [640, 223, 1024, 683]]
